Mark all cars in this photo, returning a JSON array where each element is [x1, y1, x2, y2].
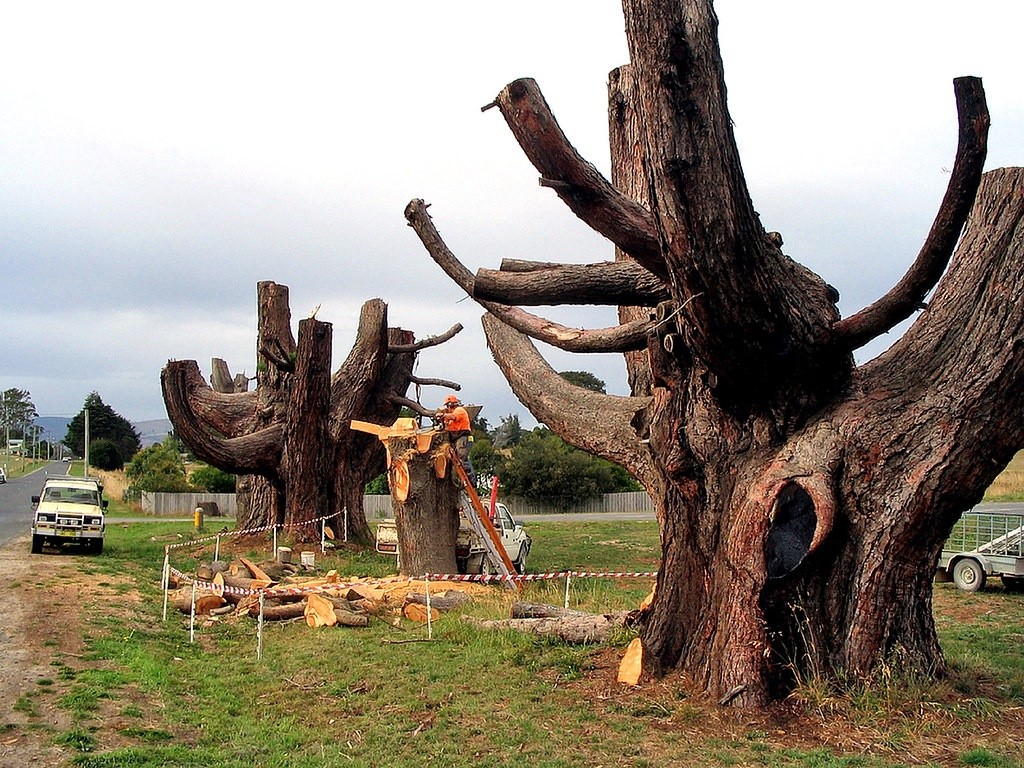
[[66, 457, 71, 460], [63, 457, 69, 463]]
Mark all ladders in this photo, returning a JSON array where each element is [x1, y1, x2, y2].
[[445, 441, 525, 593]]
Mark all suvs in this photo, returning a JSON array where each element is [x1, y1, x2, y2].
[[29, 472, 111, 555]]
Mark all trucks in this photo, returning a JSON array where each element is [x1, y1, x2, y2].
[[375, 497, 532, 577]]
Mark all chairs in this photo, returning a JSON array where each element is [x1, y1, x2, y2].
[[81, 493, 92, 501], [50, 491, 62, 499]]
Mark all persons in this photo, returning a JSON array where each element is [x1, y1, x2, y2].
[[435, 396, 479, 489]]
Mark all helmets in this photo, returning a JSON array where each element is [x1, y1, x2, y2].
[[444, 395, 458, 405]]
